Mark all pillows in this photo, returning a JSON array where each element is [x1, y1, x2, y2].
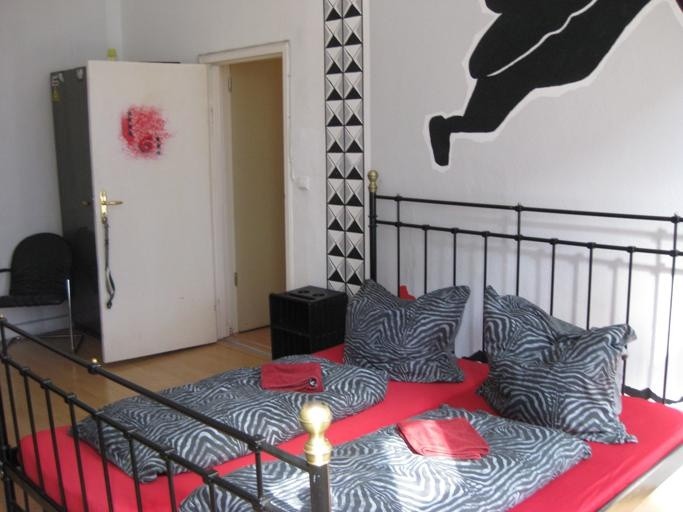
[[476, 284, 638, 445], [343, 279, 470, 383]]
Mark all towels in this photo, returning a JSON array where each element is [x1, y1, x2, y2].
[[396, 418, 490, 460], [260, 362, 324, 393]]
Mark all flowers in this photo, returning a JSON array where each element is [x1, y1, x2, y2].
[[121, 105, 172, 160]]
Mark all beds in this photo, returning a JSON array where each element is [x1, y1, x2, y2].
[[1, 342, 682, 512]]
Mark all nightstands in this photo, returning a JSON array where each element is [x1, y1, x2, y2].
[[268, 285, 347, 361]]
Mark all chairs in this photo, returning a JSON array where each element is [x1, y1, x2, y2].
[[0, 232, 84, 353]]
